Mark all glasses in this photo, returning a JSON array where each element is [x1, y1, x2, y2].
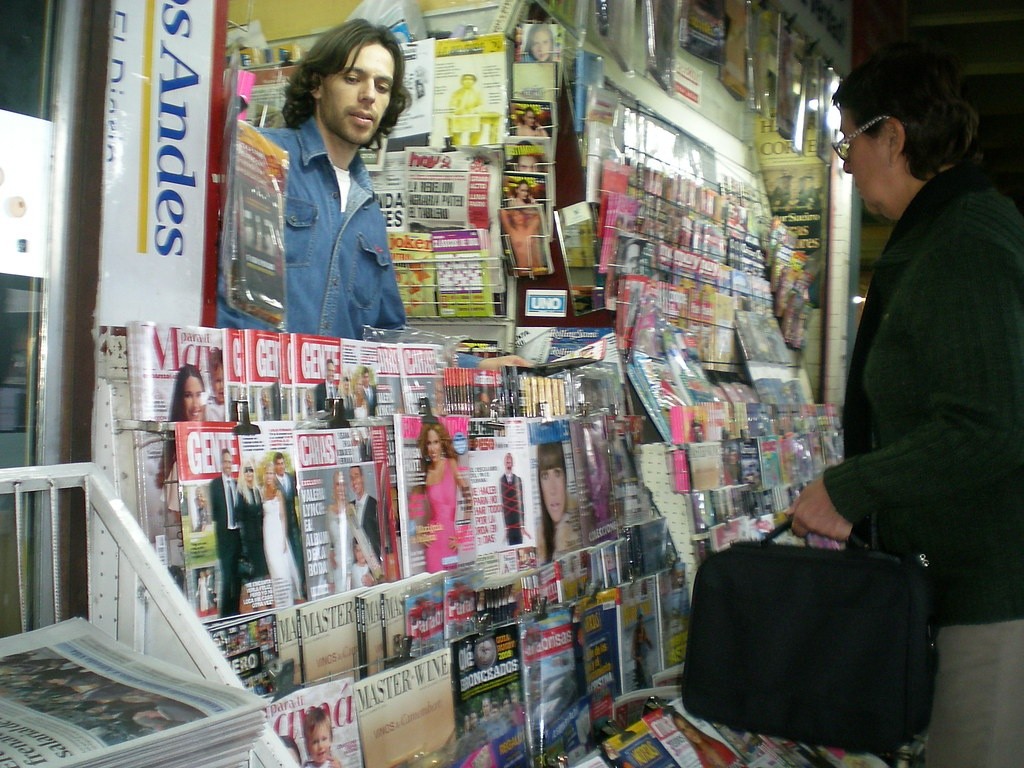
[[832, 117, 907, 162], [243, 467, 254, 473]]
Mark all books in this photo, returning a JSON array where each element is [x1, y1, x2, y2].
[[520, 23, 566, 98], [127, 33, 889, 768]]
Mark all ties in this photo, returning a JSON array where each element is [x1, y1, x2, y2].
[[226, 480, 236, 527], [329, 386, 333, 398]]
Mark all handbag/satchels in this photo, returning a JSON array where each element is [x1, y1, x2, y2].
[[680, 518, 936, 755]]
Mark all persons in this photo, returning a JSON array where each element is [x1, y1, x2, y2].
[[153, 23, 835, 768], [213, 17, 408, 342], [783, 44, 1024, 768]]
